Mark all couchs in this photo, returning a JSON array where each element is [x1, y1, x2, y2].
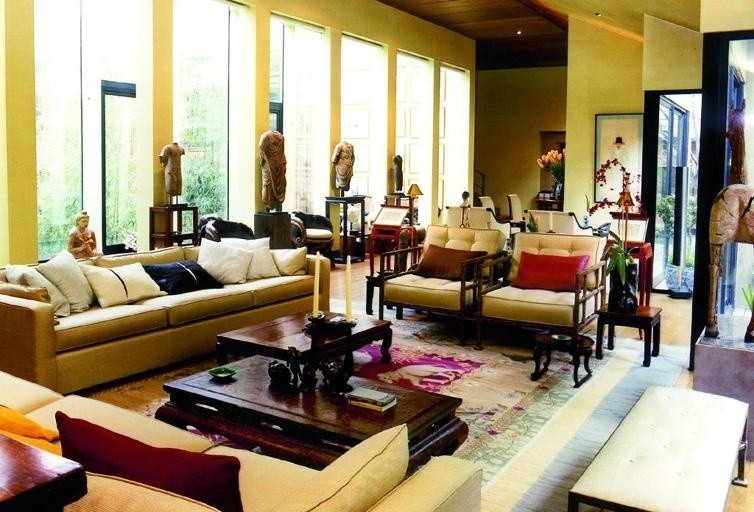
[[0, 240, 332, 395], [1, 369, 483, 512]]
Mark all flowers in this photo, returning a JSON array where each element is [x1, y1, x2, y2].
[[537, 147, 567, 183]]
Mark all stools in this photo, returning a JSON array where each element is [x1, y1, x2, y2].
[[530, 332, 595, 389]]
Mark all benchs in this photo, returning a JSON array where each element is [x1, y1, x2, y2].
[[563, 382, 748, 512]]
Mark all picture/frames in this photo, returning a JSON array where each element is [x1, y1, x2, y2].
[[594, 112, 645, 219]]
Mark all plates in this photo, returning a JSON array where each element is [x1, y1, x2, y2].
[[208, 367, 237, 379]]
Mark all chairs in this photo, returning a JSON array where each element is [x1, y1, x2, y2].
[[289, 212, 335, 269], [379, 194, 609, 365]]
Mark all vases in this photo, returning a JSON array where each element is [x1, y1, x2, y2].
[[554, 182, 564, 201]]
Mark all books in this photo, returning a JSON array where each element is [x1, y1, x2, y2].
[[344, 388, 395, 408], [347, 397, 397, 413]]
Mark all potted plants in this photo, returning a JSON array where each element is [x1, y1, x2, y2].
[[604, 231, 639, 313], [658, 193, 696, 301]]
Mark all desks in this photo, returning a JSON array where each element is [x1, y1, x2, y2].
[[597, 302, 662, 366], [604, 237, 654, 340]]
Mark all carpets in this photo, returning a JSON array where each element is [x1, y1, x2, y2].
[[82, 295, 697, 511]]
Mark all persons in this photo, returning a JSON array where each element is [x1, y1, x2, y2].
[[258, 131, 287, 211], [392, 155, 405, 193], [68, 210, 105, 260], [158, 142, 184, 195], [607, 137, 632, 194], [331, 141, 355, 191]]
